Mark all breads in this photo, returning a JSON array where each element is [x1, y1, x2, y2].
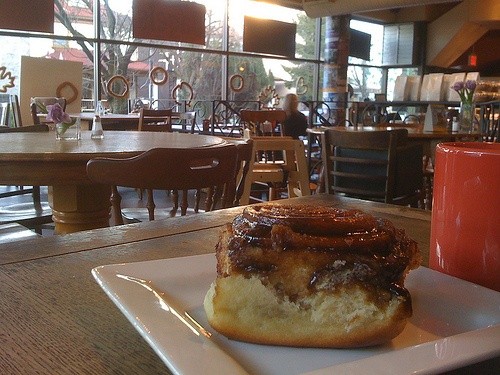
[[204, 204, 418, 348]]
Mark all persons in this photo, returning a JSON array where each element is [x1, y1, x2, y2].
[[268, 94, 308, 160]]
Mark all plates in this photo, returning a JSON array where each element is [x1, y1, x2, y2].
[[91, 253, 500, 375]]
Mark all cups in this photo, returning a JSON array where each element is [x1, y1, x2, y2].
[[428, 142, 500, 292]]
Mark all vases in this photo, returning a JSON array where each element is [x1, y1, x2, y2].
[[457, 102, 477, 142], [54, 117, 81, 141]]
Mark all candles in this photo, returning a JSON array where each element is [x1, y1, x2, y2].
[[428, 142, 500, 291]]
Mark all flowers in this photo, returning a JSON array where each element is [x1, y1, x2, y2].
[[37, 101, 76, 135], [451, 79, 477, 130]]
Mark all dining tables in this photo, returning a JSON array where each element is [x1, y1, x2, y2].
[[0, 194, 432, 375]]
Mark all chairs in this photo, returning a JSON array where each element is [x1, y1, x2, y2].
[[477, 100, 500, 142], [139, 109, 434, 211]]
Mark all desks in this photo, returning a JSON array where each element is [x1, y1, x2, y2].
[[30, 113, 180, 131], [0, 131, 229, 234], [289, 127, 490, 206]]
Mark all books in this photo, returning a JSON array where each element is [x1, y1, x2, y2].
[[0, 94, 22, 128]]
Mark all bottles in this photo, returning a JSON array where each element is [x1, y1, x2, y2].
[[91, 115, 105, 139], [244, 127, 251, 142], [444, 111, 460, 132]]
